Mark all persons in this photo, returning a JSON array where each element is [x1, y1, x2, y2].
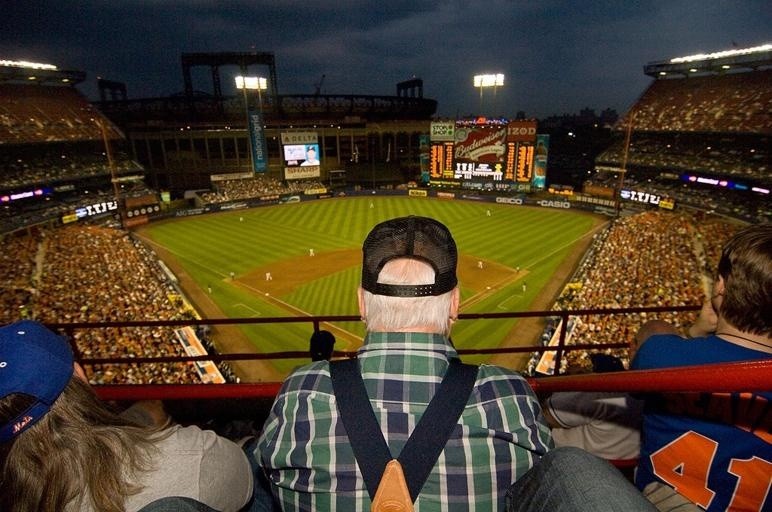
[[523, 90, 771, 399], [505, 228, 772, 511], [0, 320, 253, 510], [254, 173, 324, 202], [247, 217, 549, 511], [1, 88, 242, 386]]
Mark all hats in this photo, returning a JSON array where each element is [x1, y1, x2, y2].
[[310, 330, 335, 355], [589, 353, 623, 371], [362, 214, 457, 296], [0, 320, 74, 442]]
